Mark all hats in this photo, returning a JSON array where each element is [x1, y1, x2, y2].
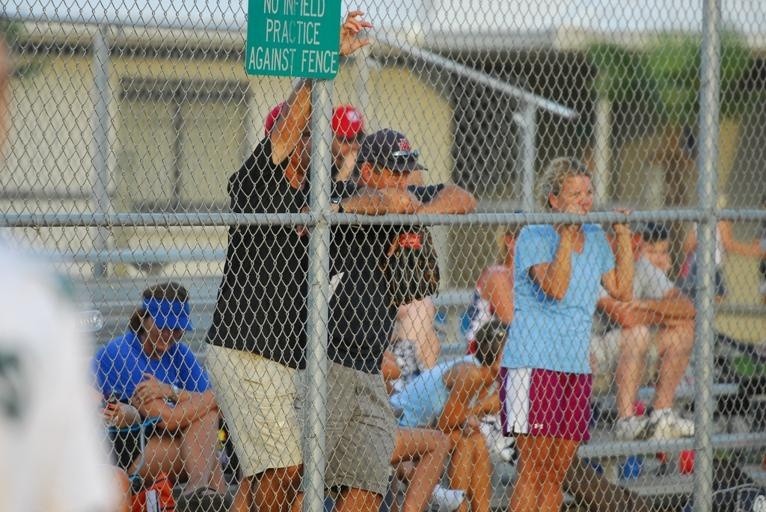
[[143, 298, 192, 330], [600, 202, 646, 236], [330, 106, 368, 141], [265, 100, 312, 134], [356, 127, 426, 171]]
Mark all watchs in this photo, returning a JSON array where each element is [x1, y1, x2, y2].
[[332, 197, 344, 213], [169, 384, 179, 400]]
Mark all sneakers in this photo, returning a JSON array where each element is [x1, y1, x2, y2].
[[648, 409, 695, 436], [613, 415, 660, 443], [426, 483, 465, 511]]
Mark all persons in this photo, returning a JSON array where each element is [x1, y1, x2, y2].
[[387, 320, 513, 510], [380, 296, 442, 396], [329, 105, 365, 186], [292, 128, 475, 512], [202, 10, 374, 512], [90, 281, 221, 511], [1, 26, 118, 511], [460, 210, 528, 354], [497, 156, 634, 512], [589, 202, 697, 442], [95, 389, 140, 511], [673, 192, 764, 312], [656, 126, 731, 305], [637, 218, 670, 276], [378, 349, 466, 511]]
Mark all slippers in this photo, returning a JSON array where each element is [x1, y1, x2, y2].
[[177, 487, 231, 512]]
